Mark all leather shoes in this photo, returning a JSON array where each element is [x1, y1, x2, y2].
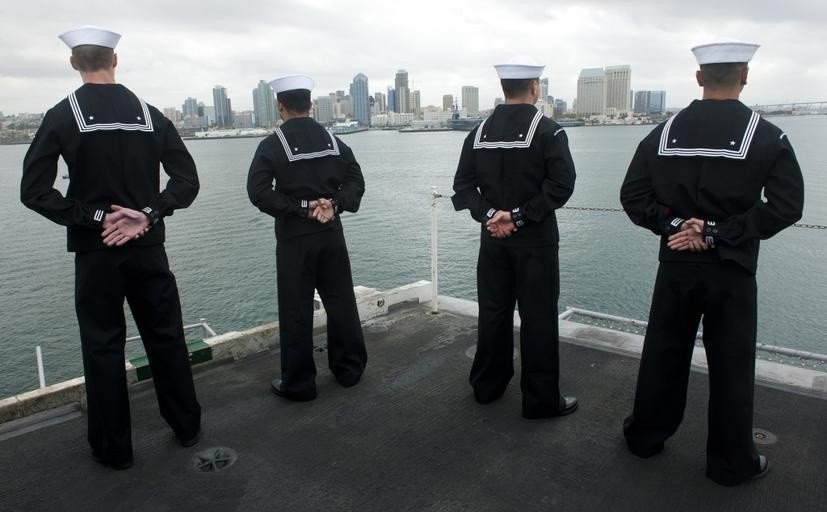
[[741, 452, 771, 480], [272, 378, 285, 399], [523, 395, 579, 420]]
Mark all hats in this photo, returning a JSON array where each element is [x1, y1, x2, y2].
[[493, 63, 547, 80], [59, 27, 122, 50], [690, 41, 760, 64], [269, 74, 316, 95]]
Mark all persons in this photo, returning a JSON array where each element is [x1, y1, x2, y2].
[[616, 37, 805, 485], [15, 25, 205, 471], [242, 75, 370, 403], [449, 61, 577, 420]]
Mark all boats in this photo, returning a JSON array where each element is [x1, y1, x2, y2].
[[381, 124, 396, 133], [554, 118, 584, 127], [398, 124, 453, 132], [448, 98, 484, 133], [330, 119, 367, 136]]
